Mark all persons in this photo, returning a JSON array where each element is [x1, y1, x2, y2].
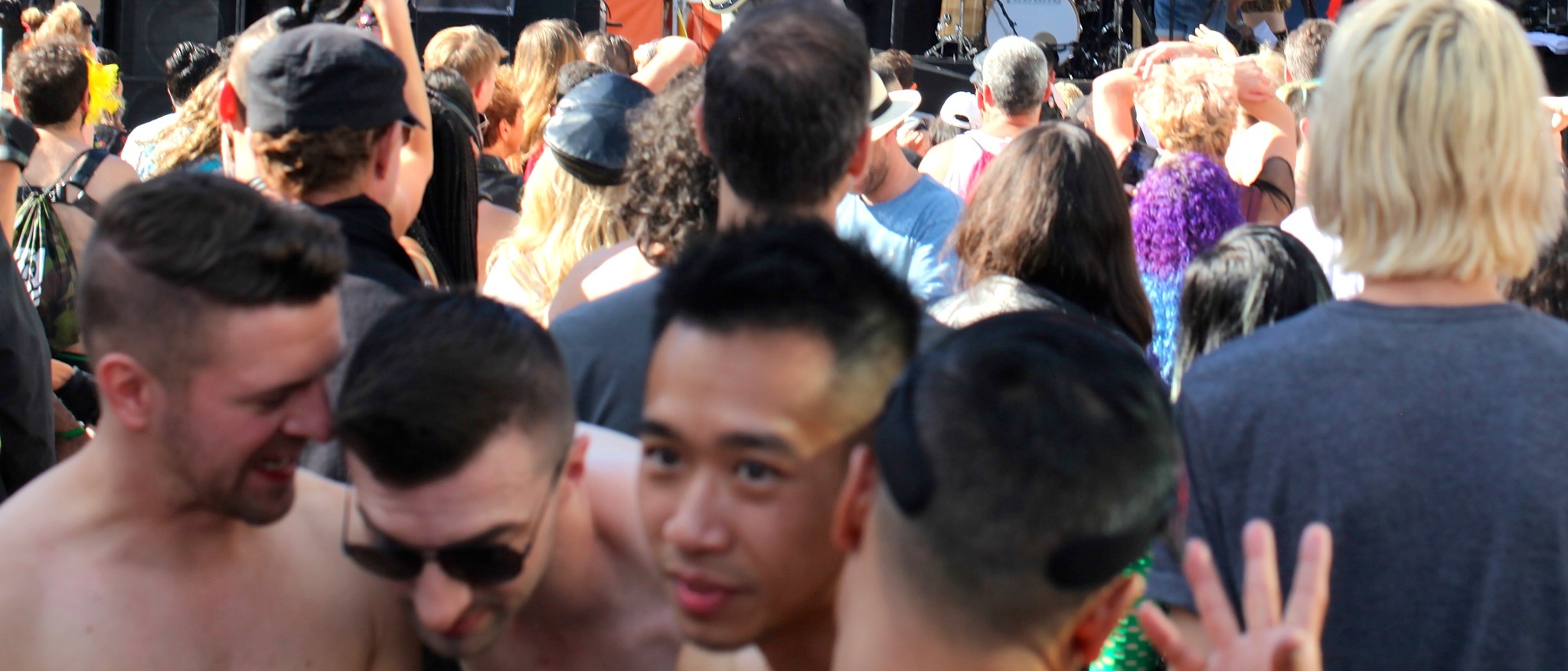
[[635, 212, 931, 671], [0, 1, 1568, 671]]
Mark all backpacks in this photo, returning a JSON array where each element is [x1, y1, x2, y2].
[[10, 145, 98, 351]]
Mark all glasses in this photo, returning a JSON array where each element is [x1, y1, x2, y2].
[[341, 446, 571, 587], [476, 112, 489, 133]]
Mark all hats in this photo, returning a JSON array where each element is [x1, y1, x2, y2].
[[542, 76, 660, 185], [247, 26, 426, 132], [941, 92, 981, 131], [865, 67, 921, 142]]
